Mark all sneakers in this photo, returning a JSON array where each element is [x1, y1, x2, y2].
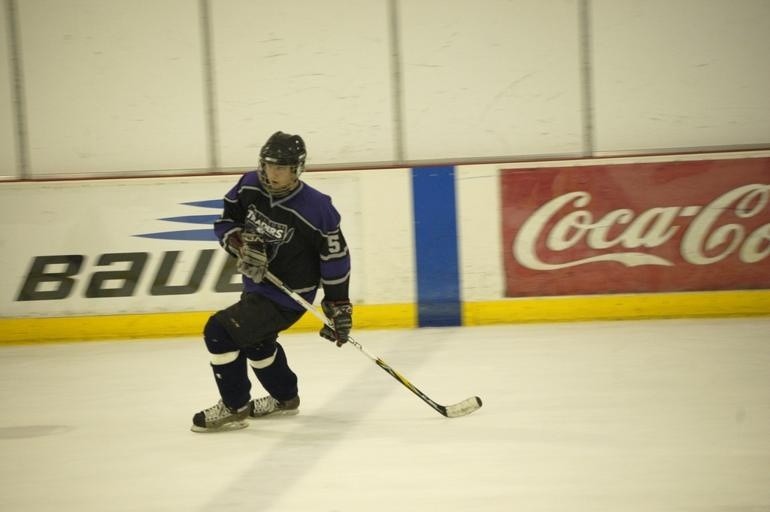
[[249, 392, 300, 416], [192, 398, 251, 428]]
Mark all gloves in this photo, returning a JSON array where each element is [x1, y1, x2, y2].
[[319, 297, 353, 348], [236, 241, 269, 283]]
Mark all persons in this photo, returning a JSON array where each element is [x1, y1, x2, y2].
[[192, 131, 352, 429]]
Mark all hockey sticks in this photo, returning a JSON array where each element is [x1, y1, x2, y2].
[[265, 271, 482, 417]]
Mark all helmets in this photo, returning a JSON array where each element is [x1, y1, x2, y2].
[[256, 130, 306, 196]]
